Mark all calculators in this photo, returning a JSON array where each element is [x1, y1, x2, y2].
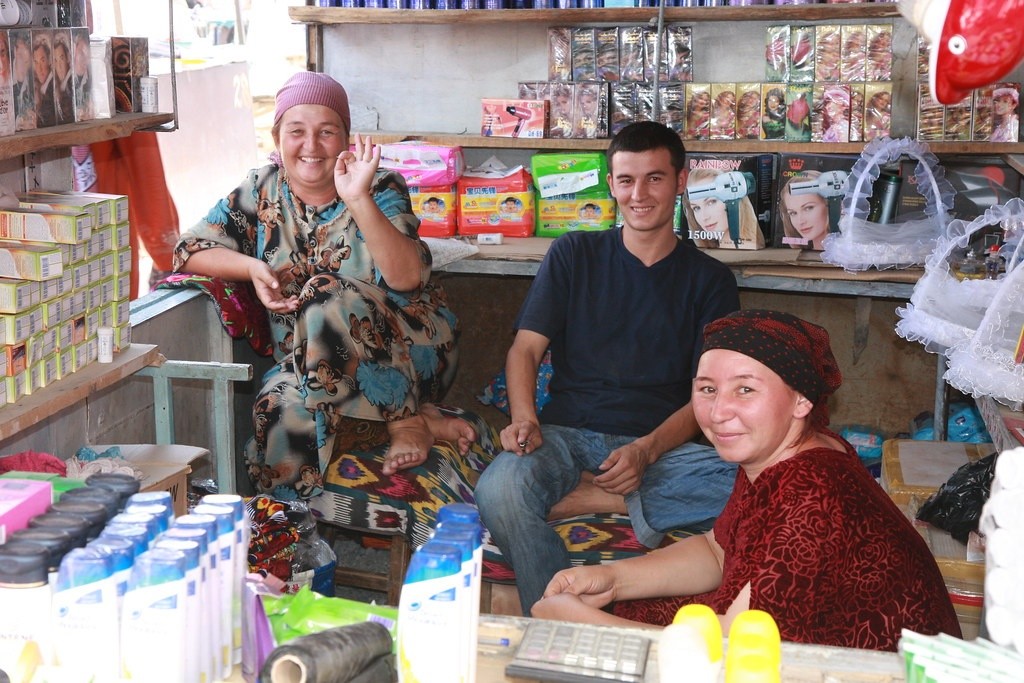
[[505, 622, 651, 683]]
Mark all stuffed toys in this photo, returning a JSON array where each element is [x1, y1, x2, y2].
[[896, 0, 1023, 105]]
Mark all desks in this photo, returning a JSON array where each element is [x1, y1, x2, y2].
[[215, 576, 1024, 683]]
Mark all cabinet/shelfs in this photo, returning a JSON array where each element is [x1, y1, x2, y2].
[[0, 0, 252, 441], [287, 0, 1024, 442]]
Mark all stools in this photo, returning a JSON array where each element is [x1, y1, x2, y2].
[[247, 406, 483, 607]]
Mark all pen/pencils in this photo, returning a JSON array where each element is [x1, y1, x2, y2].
[[477, 637, 509, 647]]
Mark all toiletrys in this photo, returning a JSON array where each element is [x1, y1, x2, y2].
[[0, 181, 490, 683]]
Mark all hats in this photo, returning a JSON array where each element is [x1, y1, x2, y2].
[[993, 87, 1020, 105], [823, 88, 849, 105]]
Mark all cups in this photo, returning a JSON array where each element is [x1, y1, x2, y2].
[[141, 77, 158, 114]]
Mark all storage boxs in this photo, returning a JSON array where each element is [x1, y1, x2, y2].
[[81, 444, 210, 519], [875, 438, 1003, 645]]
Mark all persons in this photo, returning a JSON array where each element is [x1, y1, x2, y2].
[[992, 89, 1019, 142], [522, 35, 760, 139], [0, 32, 93, 135], [473, 122, 739, 619], [583, 204, 598, 218], [762, 30, 891, 142], [779, 170, 831, 250], [500, 197, 518, 214], [426, 197, 441, 213], [530, 309, 963, 652], [174, 71, 476, 499], [682, 164, 766, 249]]
